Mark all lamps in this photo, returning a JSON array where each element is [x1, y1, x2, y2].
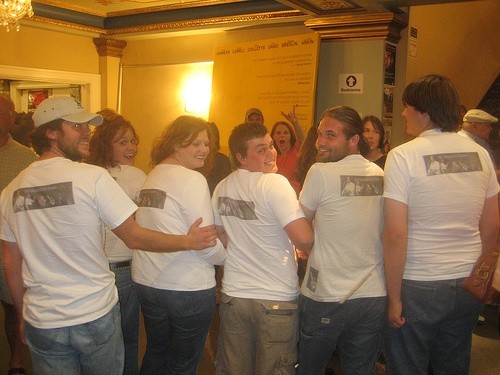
[[0, 0, 34, 35]]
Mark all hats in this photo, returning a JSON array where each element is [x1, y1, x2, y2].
[[32, 95, 103, 128], [463, 109, 498, 123], [245, 108, 263, 118]]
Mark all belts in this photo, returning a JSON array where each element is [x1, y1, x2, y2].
[[109, 261, 129, 269]]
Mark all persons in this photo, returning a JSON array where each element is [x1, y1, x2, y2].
[[456, 109, 500, 179], [212, 122, 314, 375], [245, 108, 265, 127], [0, 94, 218, 375], [192, 121, 233, 199], [296, 104, 387, 375], [86, 109, 147, 375], [131, 116, 227, 375], [383, 74, 500, 375], [271, 105, 304, 199], [0, 93, 42, 375], [361, 115, 387, 172]]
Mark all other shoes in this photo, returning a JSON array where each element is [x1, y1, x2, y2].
[[478, 303, 500, 330]]
